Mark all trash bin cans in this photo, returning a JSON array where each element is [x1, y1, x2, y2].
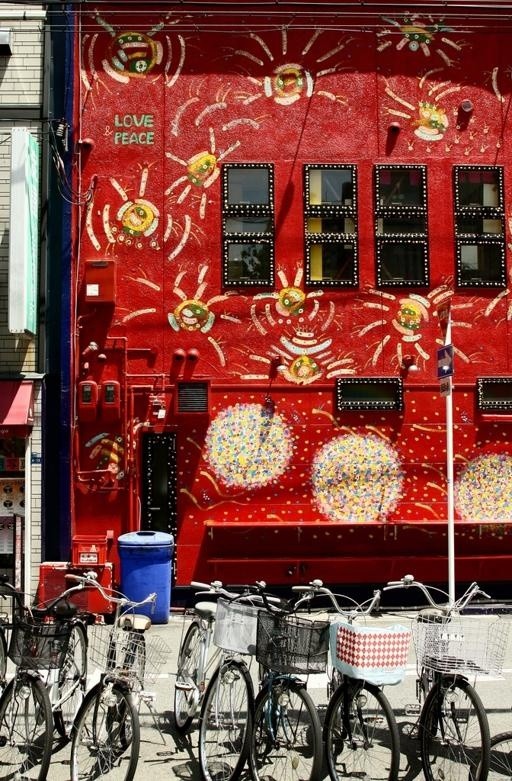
[[117, 531, 175, 624]]
